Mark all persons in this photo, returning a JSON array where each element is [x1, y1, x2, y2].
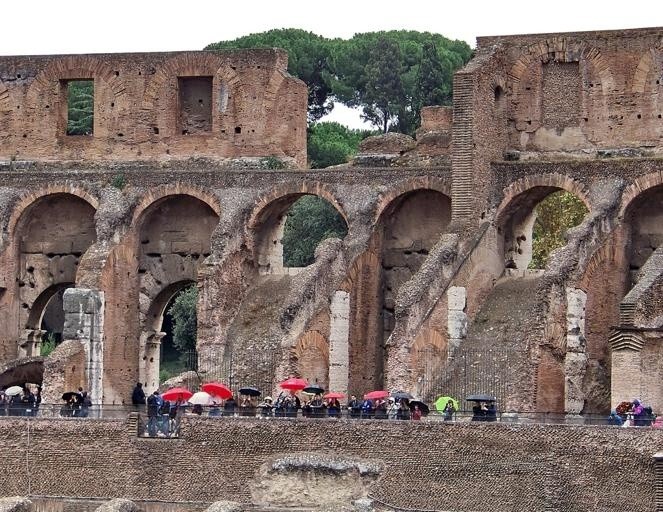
[[147, 391, 410, 437], [472, 401, 486, 421], [609, 399, 663, 426], [481, 401, 497, 421], [443, 400, 456, 421], [411, 405, 421, 420], [60, 387, 92, 416], [132, 383, 146, 406], [0, 387, 42, 416]]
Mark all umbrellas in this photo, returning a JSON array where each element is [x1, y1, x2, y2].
[[303, 386, 325, 394], [162, 387, 192, 400], [238, 387, 261, 397], [436, 396, 459, 414], [409, 401, 429, 417], [202, 383, 232, 400], [280, 379, 305, 390], [467, 394, 495, 402]]
